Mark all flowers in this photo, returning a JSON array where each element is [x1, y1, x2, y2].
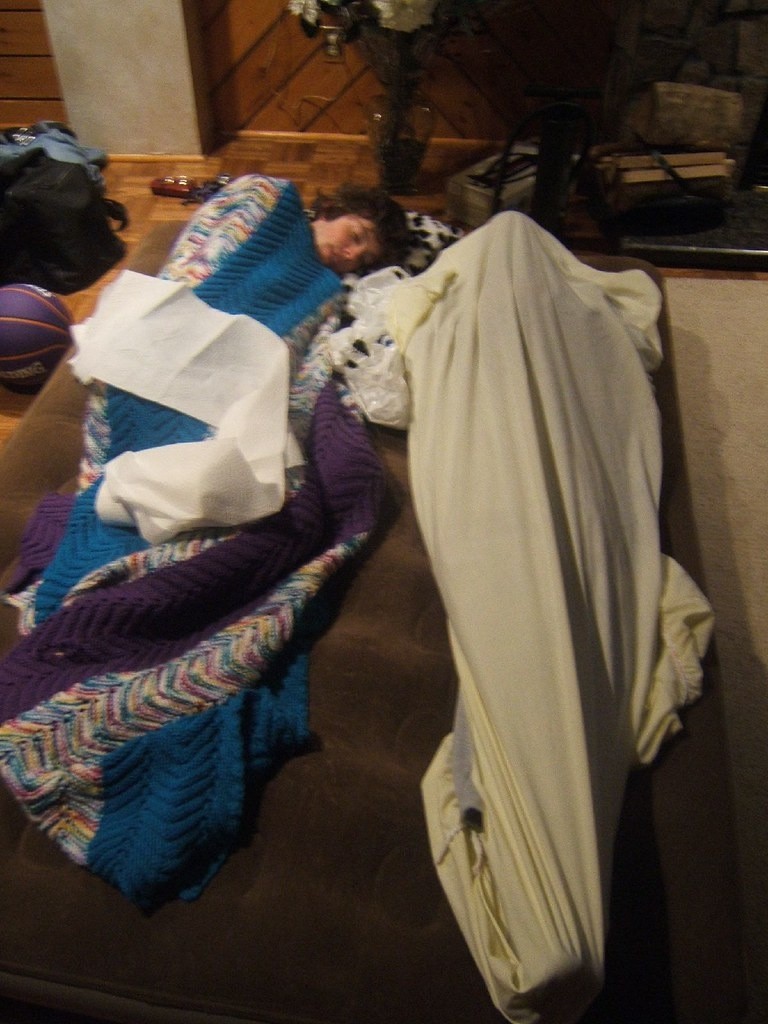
[[284, 0, 437, 39]]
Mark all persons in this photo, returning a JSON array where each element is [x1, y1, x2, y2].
[[57, 173, 411, 684]]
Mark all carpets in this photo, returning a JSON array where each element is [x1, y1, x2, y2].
[[1, 215, 767, 1024]]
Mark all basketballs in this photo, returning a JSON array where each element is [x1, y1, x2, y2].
[[0, 284, 76, 395]]
